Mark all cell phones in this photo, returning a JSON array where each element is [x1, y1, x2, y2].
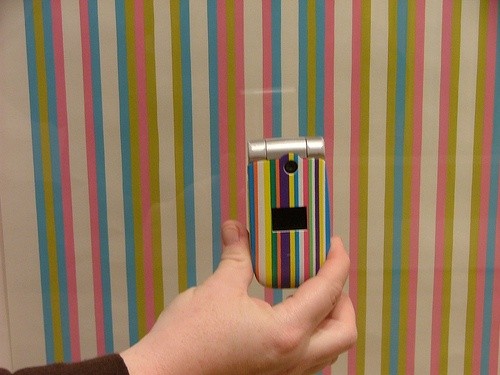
[[248, 135, 331, 289]]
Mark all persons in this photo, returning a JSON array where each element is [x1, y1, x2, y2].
[[0, 219, 360, 375]]
